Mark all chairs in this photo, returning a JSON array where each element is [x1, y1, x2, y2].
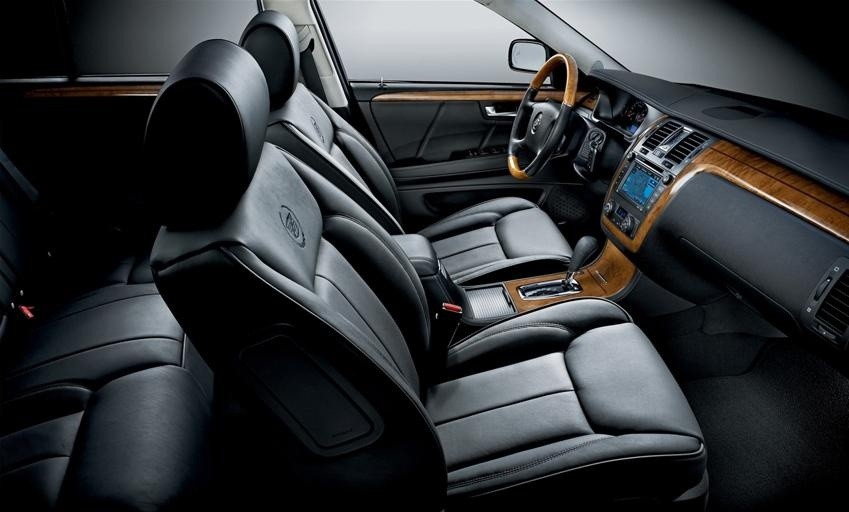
[[142, 37, 711, 509], [234, 10, 579, 287], [0, 152, 218, 509]]
